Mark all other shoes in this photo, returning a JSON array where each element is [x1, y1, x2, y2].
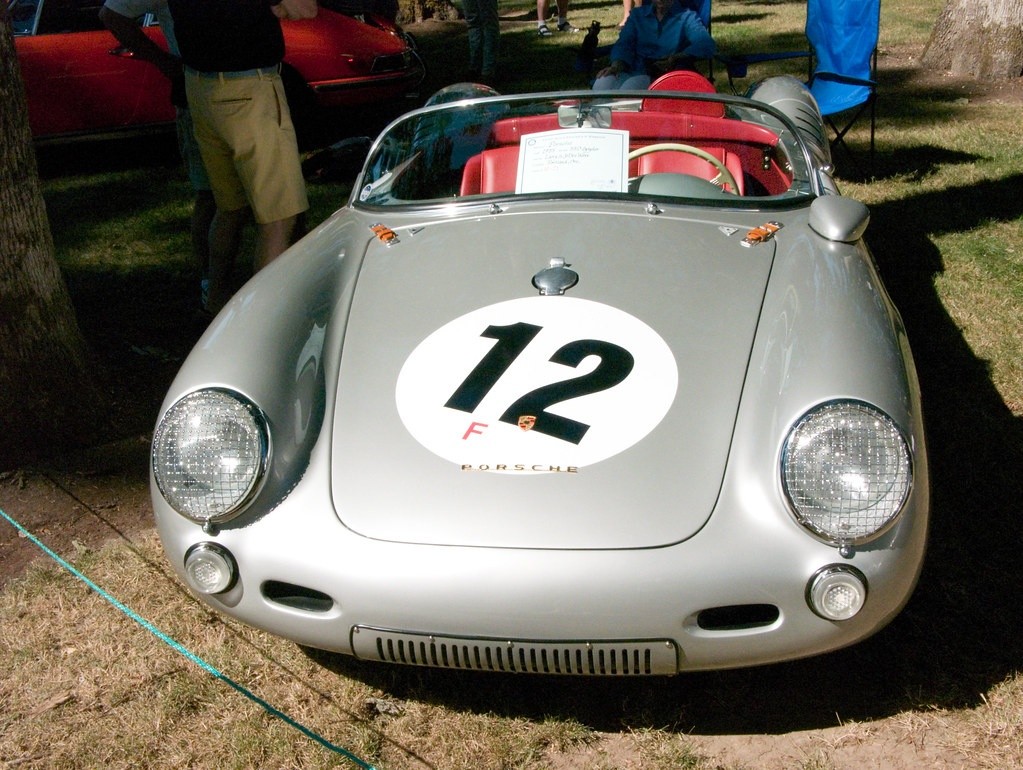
[[202, 279, 216, 312]]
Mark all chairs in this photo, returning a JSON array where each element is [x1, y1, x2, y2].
[[727, 0, 881, 163], [461, 70, 745, 197], [574, 0, 715, 88]]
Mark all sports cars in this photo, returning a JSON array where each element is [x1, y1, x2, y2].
[[150, 70, 929, 675]]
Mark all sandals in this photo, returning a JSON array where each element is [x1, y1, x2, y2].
[[537, 25, 555, 38], [557, 22, 579, 32]]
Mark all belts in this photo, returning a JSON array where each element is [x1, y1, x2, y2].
[[184, 63, 280, 79]]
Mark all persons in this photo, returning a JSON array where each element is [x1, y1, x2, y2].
[[96, 0, 215, 274], [169, 0, 319, 315], [536, 0, 574, 35], [592, 0, 718, 90], [462, 0, 500, 78]]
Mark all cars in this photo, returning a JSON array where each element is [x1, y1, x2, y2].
[[2, 0, 424, 151]]
